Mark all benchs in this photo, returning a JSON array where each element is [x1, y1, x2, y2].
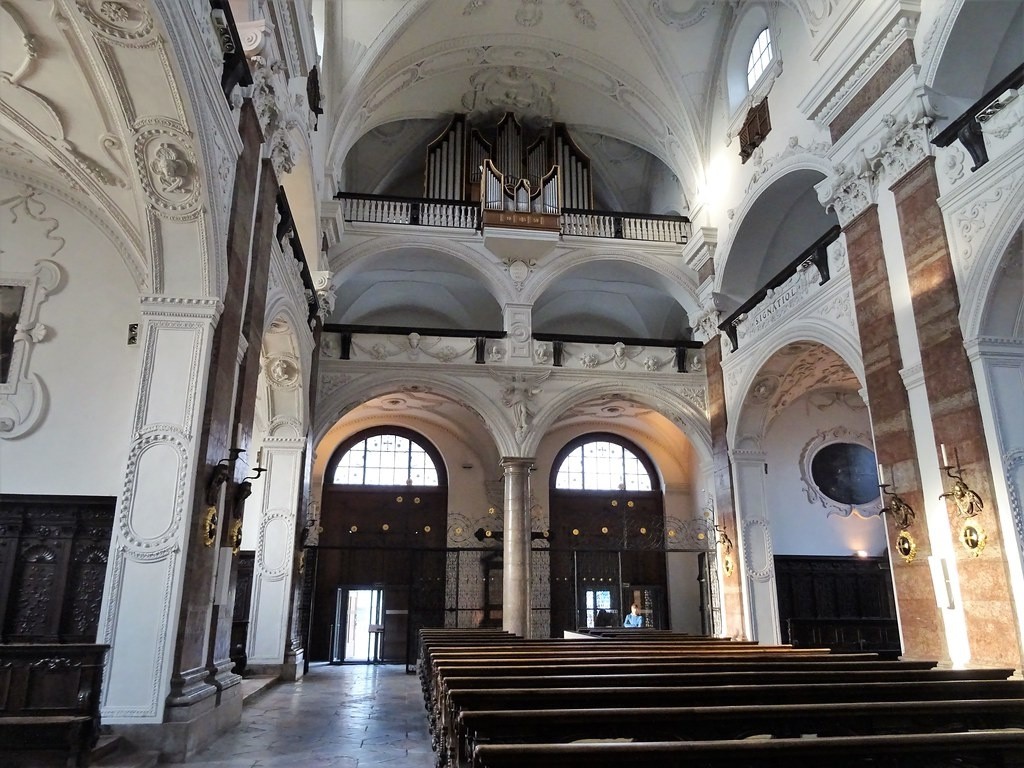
[[415, 628, 1024, 768]]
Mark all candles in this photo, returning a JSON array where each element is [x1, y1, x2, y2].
[[236, 423, 242, 448], [258, 446, 264, 468], [878, 463, 885, 484], [940, 443, 949, 466]]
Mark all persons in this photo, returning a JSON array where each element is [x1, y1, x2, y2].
[[624, 604, 642, 628], [504, 372, 543, 428]]
